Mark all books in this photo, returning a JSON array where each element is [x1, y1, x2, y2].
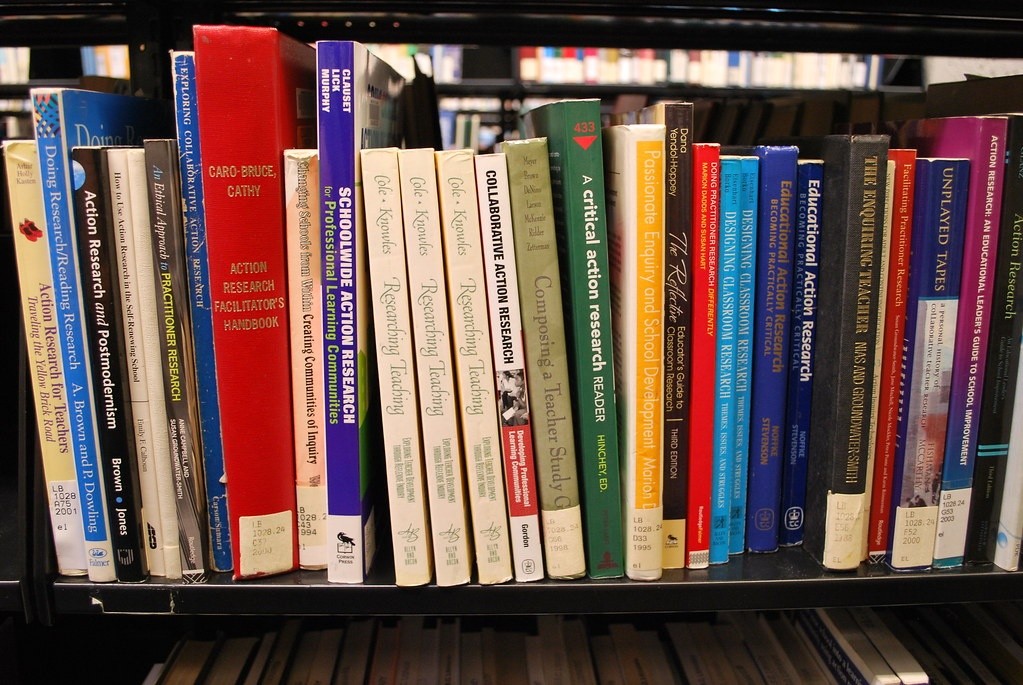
[[142, 600, 1023, 685], [1, 25, 1022, 585]]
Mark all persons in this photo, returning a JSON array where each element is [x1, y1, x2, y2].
[[502, 371, 521, 413], [512, 372, 528, 426]]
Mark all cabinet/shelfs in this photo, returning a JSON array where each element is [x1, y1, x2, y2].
[[0, 0, 1023, 635]]
